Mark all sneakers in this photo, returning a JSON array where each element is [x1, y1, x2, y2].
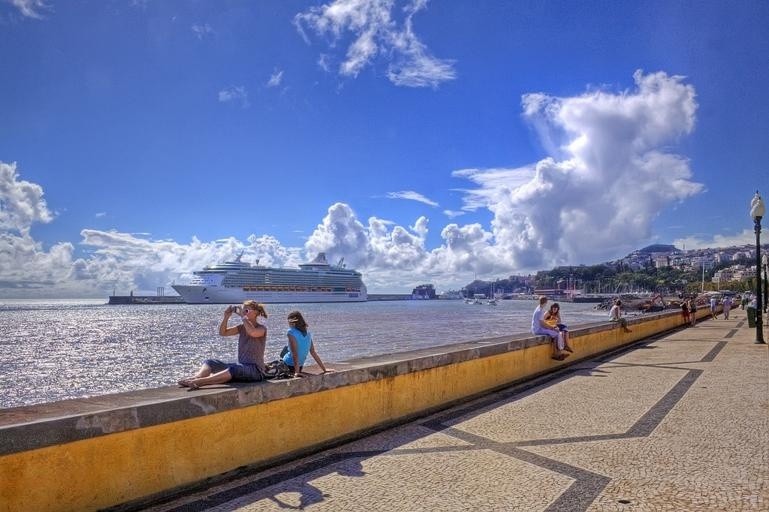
[[551, 346, 573, 361]]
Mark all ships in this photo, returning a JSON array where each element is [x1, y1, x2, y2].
[[169, 247, 370, 304]]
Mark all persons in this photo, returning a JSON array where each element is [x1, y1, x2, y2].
[[609, 299, 633, 332], [178, 300, 266, 389], [709, 295, 721, 320], [722, 296, 732, 320], [680, 297, 697, 327], [266, 311, 334, 377], [532, 296, 574, 360], [738, 294, 756, 310]]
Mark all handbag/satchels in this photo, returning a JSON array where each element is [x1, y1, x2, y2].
[[280, 346, 288, 358]]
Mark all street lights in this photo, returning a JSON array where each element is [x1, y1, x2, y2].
[[748, 189, 766, 346]]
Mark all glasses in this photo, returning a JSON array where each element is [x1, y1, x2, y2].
[[245, 309, 255, 313]]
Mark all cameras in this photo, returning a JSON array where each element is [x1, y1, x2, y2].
[[231, 306, 238, 313]]
